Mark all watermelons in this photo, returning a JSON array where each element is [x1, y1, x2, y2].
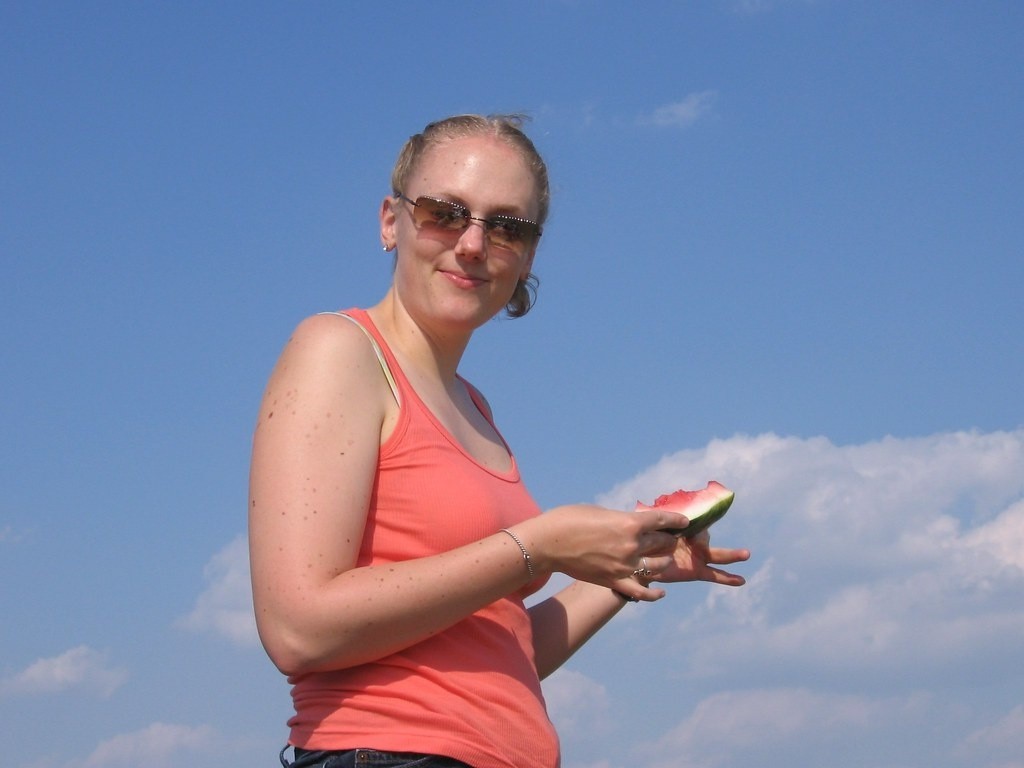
[[631, 479, 734, 538]]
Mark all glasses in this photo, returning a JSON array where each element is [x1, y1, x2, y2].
[[385, 189, 548, 264]]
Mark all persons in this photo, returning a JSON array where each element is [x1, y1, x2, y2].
[[249, 115, 750, 768]]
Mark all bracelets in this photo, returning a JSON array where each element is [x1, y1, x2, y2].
[[615, 584, 649, 603], [498, 528, 533, 579]]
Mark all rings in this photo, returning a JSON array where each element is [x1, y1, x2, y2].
[[633, 556, 651, 577]]
